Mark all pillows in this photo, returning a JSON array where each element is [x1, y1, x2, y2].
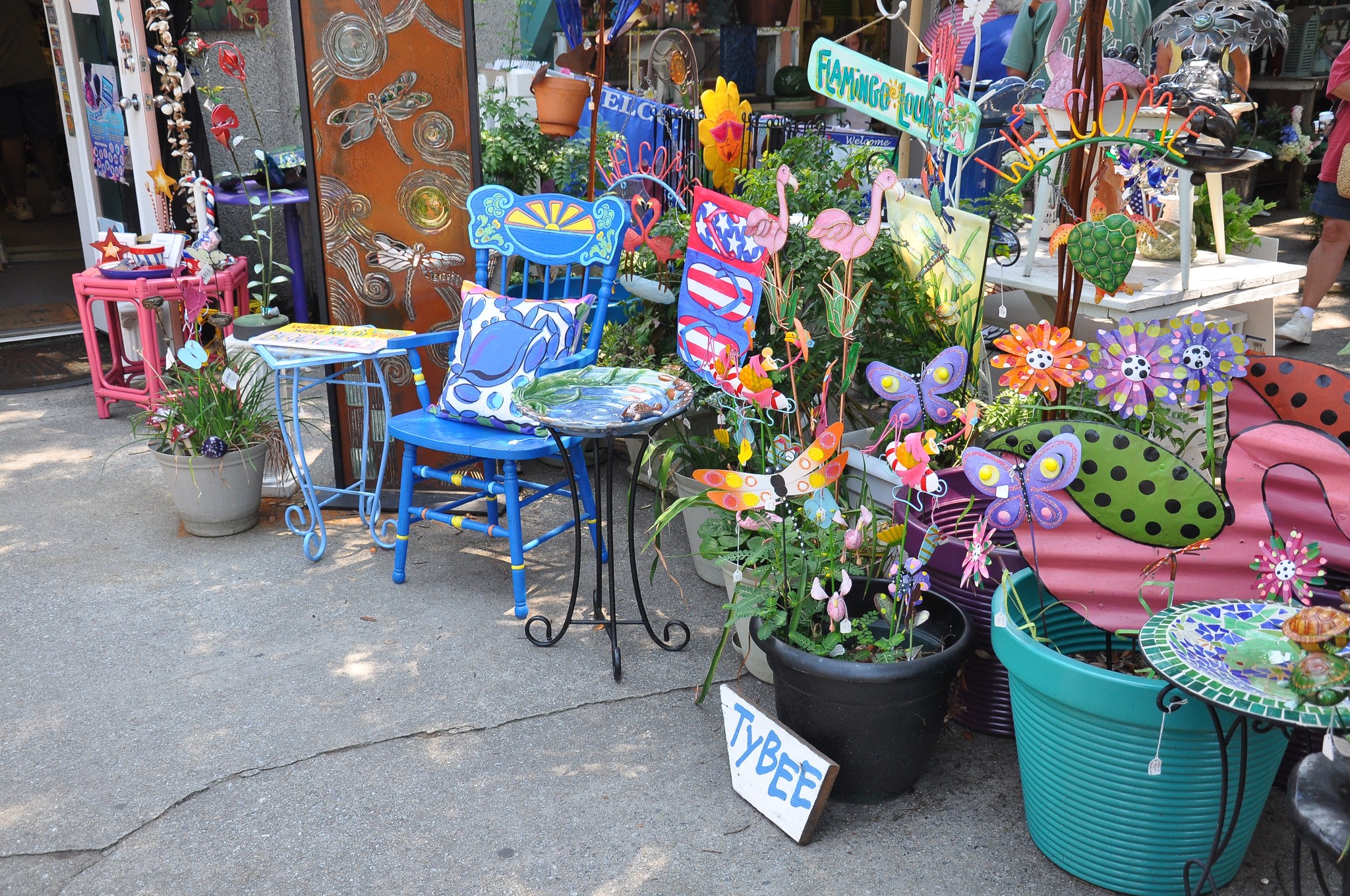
[[421, 279, 597, 438]]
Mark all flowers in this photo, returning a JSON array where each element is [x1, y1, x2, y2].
[[714, 506, 905, 663], [179, 32, 294, 318], [1234, 101, 1322, 172]]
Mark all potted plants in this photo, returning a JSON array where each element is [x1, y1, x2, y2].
[[582, 127, 1200, 736], [98, 344, 336, 538]]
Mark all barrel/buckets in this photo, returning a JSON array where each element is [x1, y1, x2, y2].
[[532, 76, 590, 136]]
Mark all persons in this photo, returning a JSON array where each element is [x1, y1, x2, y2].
[[817, 23, 872, 130], [915, 0, 1033, 148], [1275, 39, 1350, 345], [1001, 0, 1151, 186], [1155, 0, 1249, 147]]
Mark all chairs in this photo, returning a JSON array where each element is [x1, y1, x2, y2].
[[386, 184, 630, 620]]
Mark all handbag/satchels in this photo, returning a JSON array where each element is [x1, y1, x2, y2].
[[1336, 143, 1350, 198]]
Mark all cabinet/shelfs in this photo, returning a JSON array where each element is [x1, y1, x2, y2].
[[552, 1, 892, 144]]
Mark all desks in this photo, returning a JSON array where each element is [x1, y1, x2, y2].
[[1021, 102, 1259, 290], [248, 323, 407, 561], [983, 218, 1309, 489], [212, 178, 311, 324], [72, 256, 251, 429]]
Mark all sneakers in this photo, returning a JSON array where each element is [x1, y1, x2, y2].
[[1275, 310, 1312, 344]]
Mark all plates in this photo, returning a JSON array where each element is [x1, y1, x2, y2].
[[511, 365, 694, 438], [96, 261, 187, 279], [1139, 599, 1350, 728]]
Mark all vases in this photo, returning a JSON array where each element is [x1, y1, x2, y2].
[[506, 274, 645, 326], [749, 573, 979, 805], [1221, 165, 1260, 205], [989, 566, 1294, 896], [533, 76, 591, 136]]
